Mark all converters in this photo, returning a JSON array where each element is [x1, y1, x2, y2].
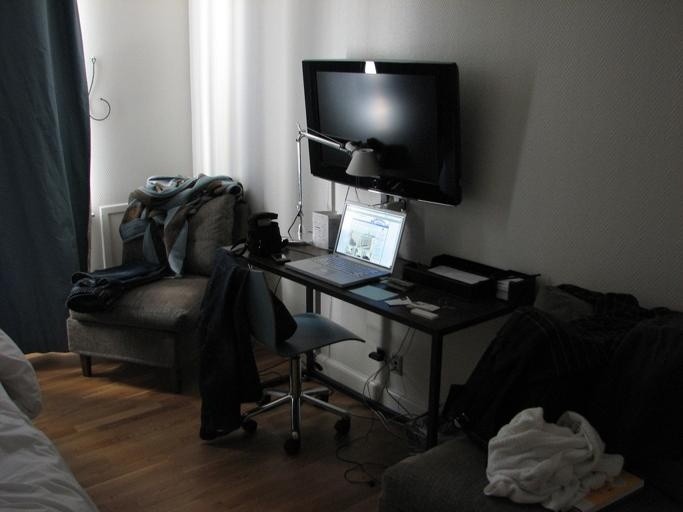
[[368, 351, 384, 363]]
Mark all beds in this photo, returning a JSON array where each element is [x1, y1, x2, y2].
[[0, 324, 101, 512]]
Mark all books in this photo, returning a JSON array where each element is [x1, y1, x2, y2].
[[571, 468, 645, 511]]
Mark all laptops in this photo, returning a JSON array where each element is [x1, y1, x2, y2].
[[285, 199, 408, 289]]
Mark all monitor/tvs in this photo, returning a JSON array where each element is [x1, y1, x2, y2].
[[302, 59, 462, 208]]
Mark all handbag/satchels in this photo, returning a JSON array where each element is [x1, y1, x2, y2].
[[247, 212, 282, 256]]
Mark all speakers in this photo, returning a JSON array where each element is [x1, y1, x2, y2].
[[311, 209, 341, 253]]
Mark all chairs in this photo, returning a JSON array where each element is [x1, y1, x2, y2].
[[67, 187, 251, 394], [381, 284, 682, 512], [210, 247, 366, 458]]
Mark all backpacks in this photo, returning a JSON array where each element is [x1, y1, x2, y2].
[[441, 307, 587, 455]]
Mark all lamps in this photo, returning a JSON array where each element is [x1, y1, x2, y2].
[[286, 122, 385, 246]]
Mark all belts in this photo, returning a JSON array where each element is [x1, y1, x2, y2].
[[65, 286, 112, 307]]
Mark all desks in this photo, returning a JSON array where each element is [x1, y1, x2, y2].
[[223, 241, 541, 450]]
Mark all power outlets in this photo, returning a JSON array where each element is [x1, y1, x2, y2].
[[376, 348, 385, 363], [390, 353, 402, 376]]
